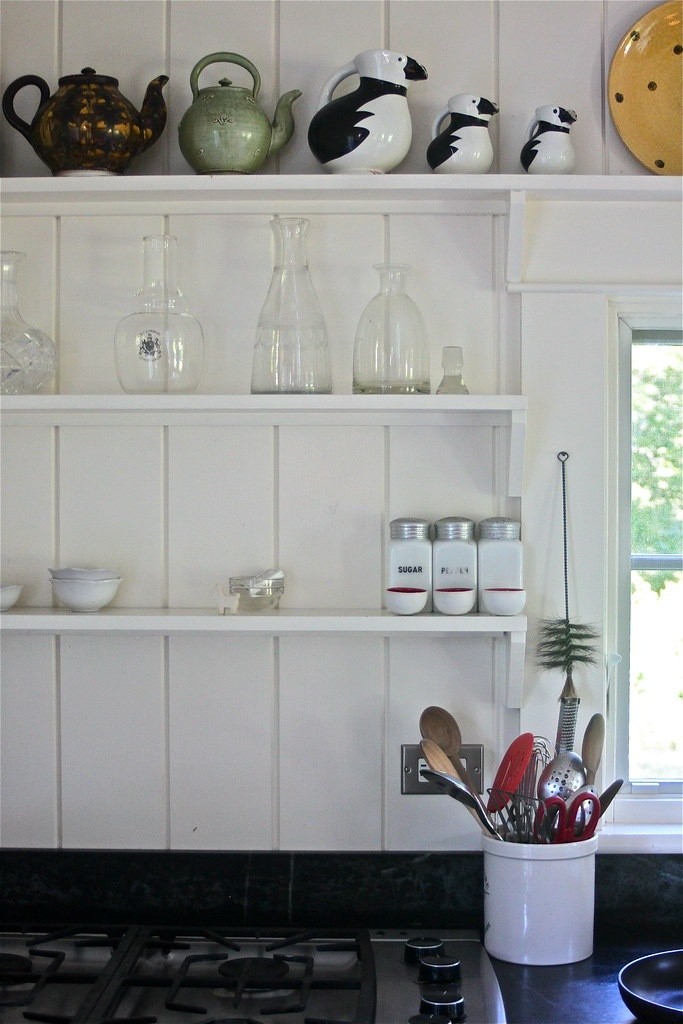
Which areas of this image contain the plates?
[606,0,683,175]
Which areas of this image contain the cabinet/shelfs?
[0,156,530,632]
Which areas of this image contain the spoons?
[419,769,505,842]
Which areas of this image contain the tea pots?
[177,51,303,175]
[1,67,170,176]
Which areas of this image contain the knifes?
[597,778,623,817]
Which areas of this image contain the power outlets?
[400,743,485,795]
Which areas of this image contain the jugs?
[425,93,499,175]
[308,50,430,178]
[519,105,577,175]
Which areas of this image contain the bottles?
[250,216,334,395]
[0,249,58,395]
[477,517,523,614]
[112,233,204,396]
[431,517,478,614]
[436,346,470,395]
[351,261,434,396]
[386,518,433,615]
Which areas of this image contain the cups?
[480,830,600,966]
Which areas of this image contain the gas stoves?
[0,922,509,1024]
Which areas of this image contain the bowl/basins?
[47,567,124,614]
[482,588,527,617]
[617,949,683,1024]
[433,588,475,615]
[229,575,284,613]
[385,587,428,616]
[0,581,23,612]
